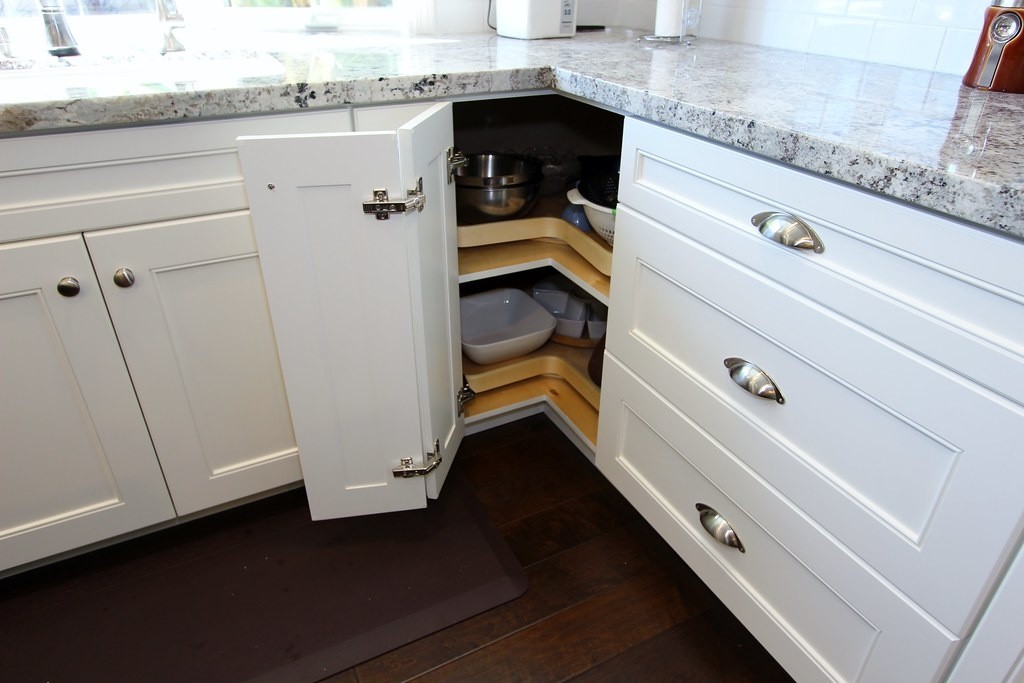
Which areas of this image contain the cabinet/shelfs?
[0,107,357,582]
[237,95,625,521]
[594,116,1024,683]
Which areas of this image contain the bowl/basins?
[455,150,545,221]
[562,203,590,233]
[459,286,559,366]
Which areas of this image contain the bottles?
[39,0,80,57]
